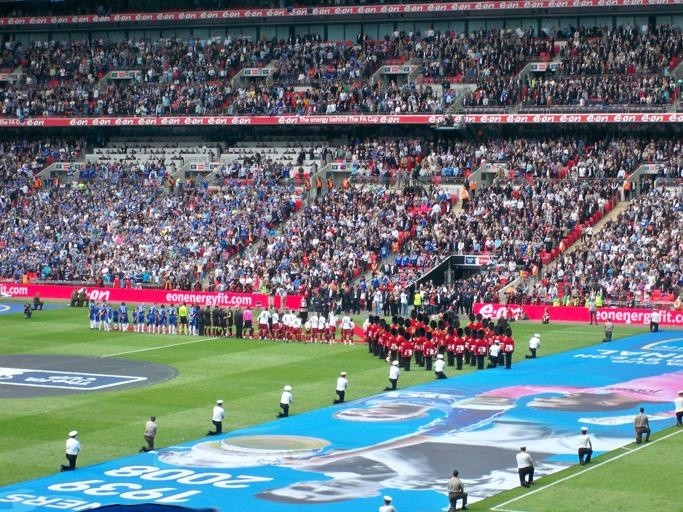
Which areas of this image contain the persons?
[156,445,282,470]
[1,0,682,113]
[138,416,157,453]
[515,446,533,488]
[634,407,650,444]
[331,371,348,404]
[206,400,225,435]
[379,495,396,512]
[478,372,682,427]
[303,386,517,461]
[0,135,683,369]
[59,430,80,471]
[276,384,292,418]
[672,391,682,427]
[577,427,592,465]
[432,354,447,379]
[446,471,467,512]
[382,360,399,391]
[247,423,629,502]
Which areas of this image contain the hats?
[384,391,683,501]
[68,371,347,438]
[369,310,541,365]
